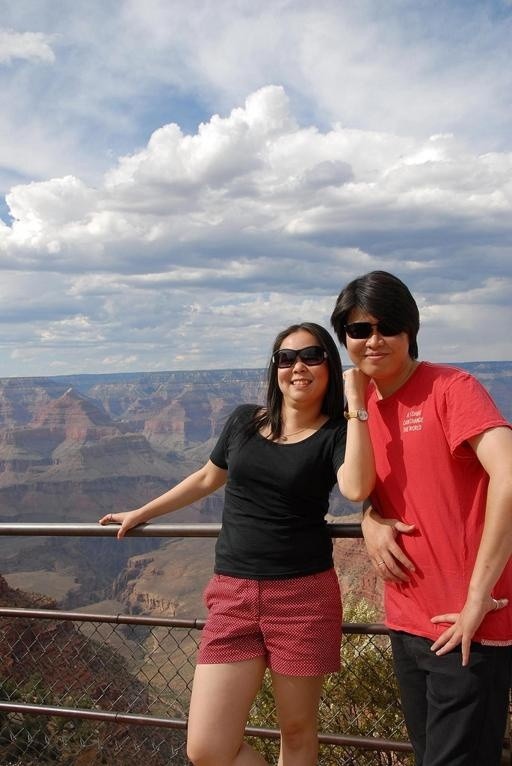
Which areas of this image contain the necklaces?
[278,416,319,441]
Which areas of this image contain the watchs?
[343,407,368,422]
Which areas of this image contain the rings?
[493,599,501,612]
[378,561,384,566]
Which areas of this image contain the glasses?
[344,320,405,339]
[273,345,330,369]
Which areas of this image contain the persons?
[331,270,511,766]
[98,321,377,765]
